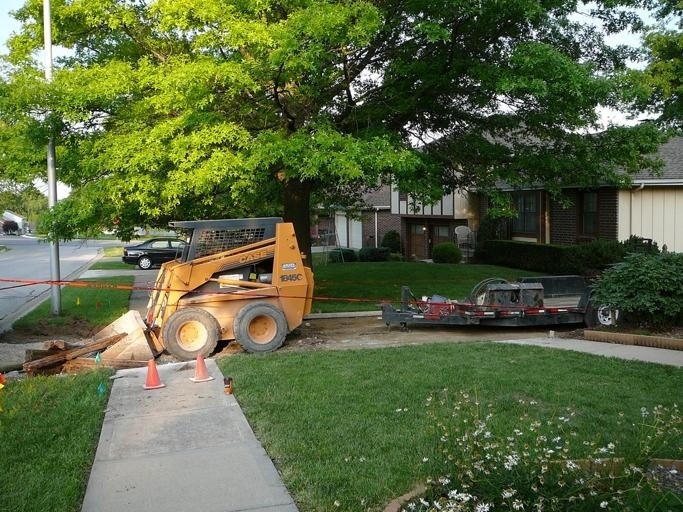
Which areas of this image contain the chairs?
[455,226,472,248]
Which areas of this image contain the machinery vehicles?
[83,215,320,369]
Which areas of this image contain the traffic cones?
[141,357,165,391]
[188,353,216,385]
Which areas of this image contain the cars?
[122,237,190,270]
[100,228,119,235]
[310,228,334,246]
[130,227,146,240]
